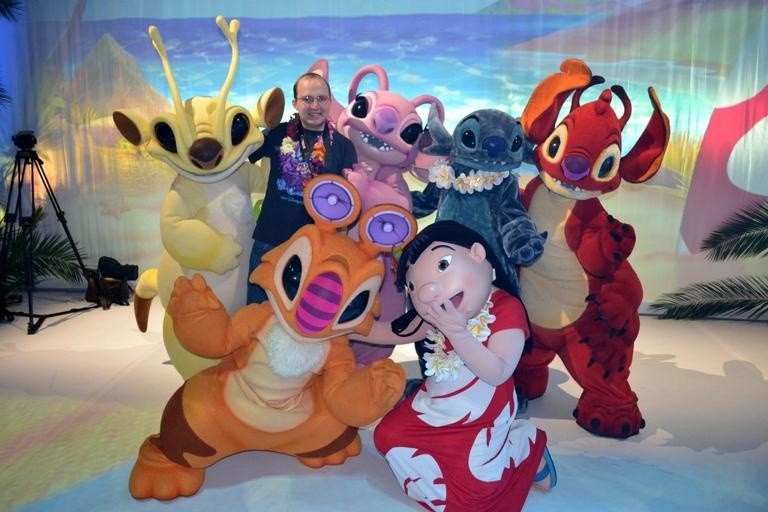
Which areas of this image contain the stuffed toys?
[126,170,422,501]
[407,102,549,294]
[311,58,454,375]
[498,57,671,438]
[110,16,287,384]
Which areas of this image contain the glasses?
[296,96,329,105]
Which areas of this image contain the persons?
[245,72,360,305]
[372,218,557,511]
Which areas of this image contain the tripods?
[0,149,105,334]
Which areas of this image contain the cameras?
[12,131,37,148]
